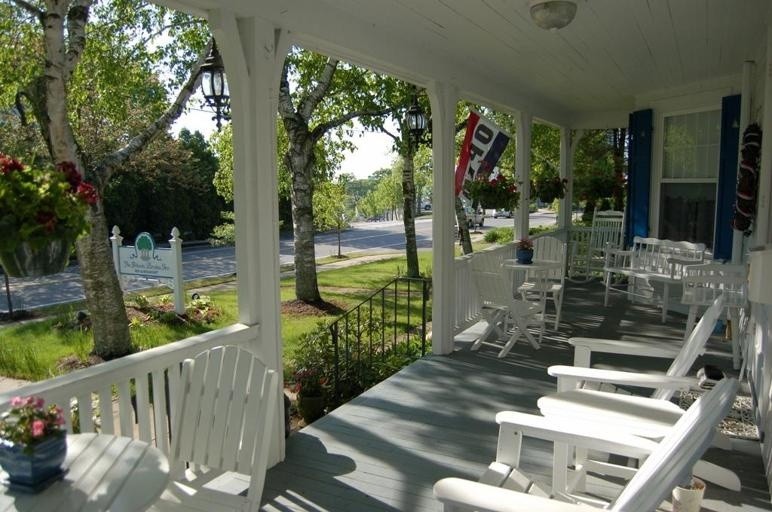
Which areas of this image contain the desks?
[0,431,171,512]
[537,386,688,498]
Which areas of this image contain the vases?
[0,233,68,278]
[0,432,71,494]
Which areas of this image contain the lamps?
[525,0,578,32]
[407,94,430,152]
[198,36,232,132]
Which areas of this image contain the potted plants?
[530,176,570,203]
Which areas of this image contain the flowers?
[0,394,67,455]
[0,151,100,259]
[462,162,521,212]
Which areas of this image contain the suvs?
[458,205,484,229]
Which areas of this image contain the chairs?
[536,286,733,467]
[430,376,743,512]
[471,205,749,371]
[140,342,281,512]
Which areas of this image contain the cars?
[416,197,433,210]
[492,209,512,219]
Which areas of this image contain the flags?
[455,106,513,208]
